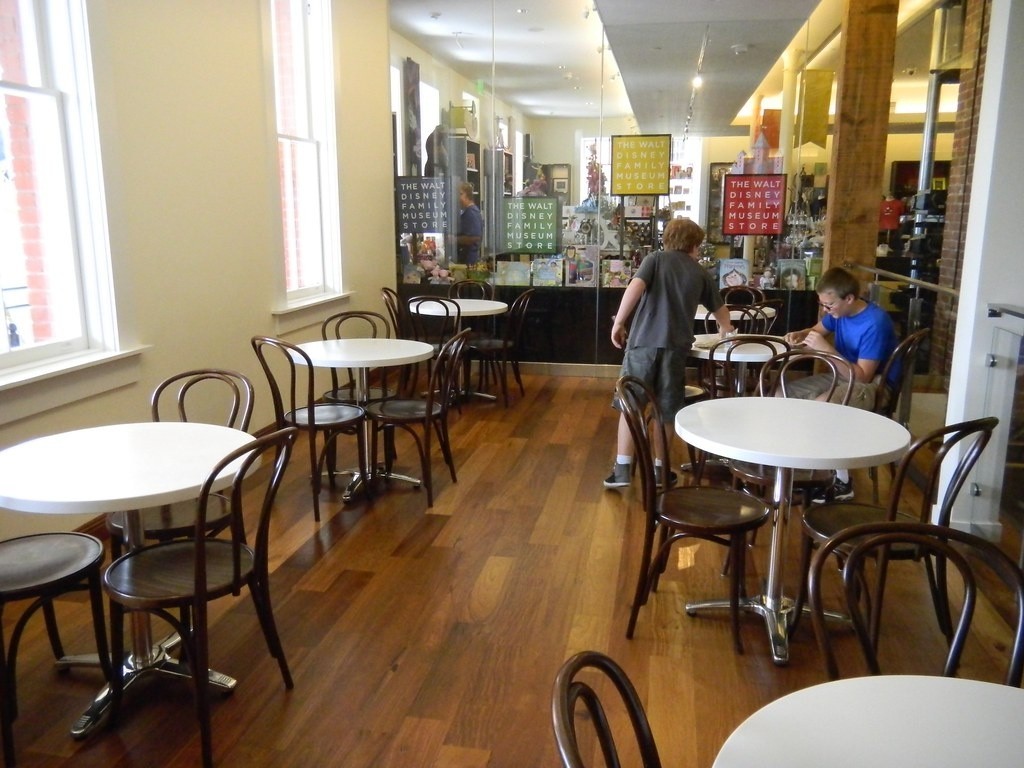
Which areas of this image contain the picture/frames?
[552,177,568,193]
[933,178,946,190]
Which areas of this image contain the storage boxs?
[804,258,822,276]
[806,275,820,291]
[778,258,806,291]
[716,258,749,290]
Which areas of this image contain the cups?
[878,245,888,257]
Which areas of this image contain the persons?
[445,182,483,262]
[783,267,800,290]
[539,261,559,272]
[809,194,827,215]
[876,194,904,229]
[772,266,899,506]
[604,217,735,488]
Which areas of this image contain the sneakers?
[811,477,855,505]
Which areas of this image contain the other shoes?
[654,465,678,488]
[603,462,632,489]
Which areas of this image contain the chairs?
[552,287,1024,768]
[0,278,536,768]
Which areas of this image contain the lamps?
[581,5,598,18]
[597,44,612,53]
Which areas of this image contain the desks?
[0,423,263,740]
[681,333,802,474]
[693,304,775,392]
[410,298,509,408]
[673,397,911,666]
[286,337,435,504]
[711,675,1024,768]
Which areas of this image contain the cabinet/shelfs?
[543,164,572,206]
[707,162,736,245]
[483,148,514,252]
[452,139,481,213]
